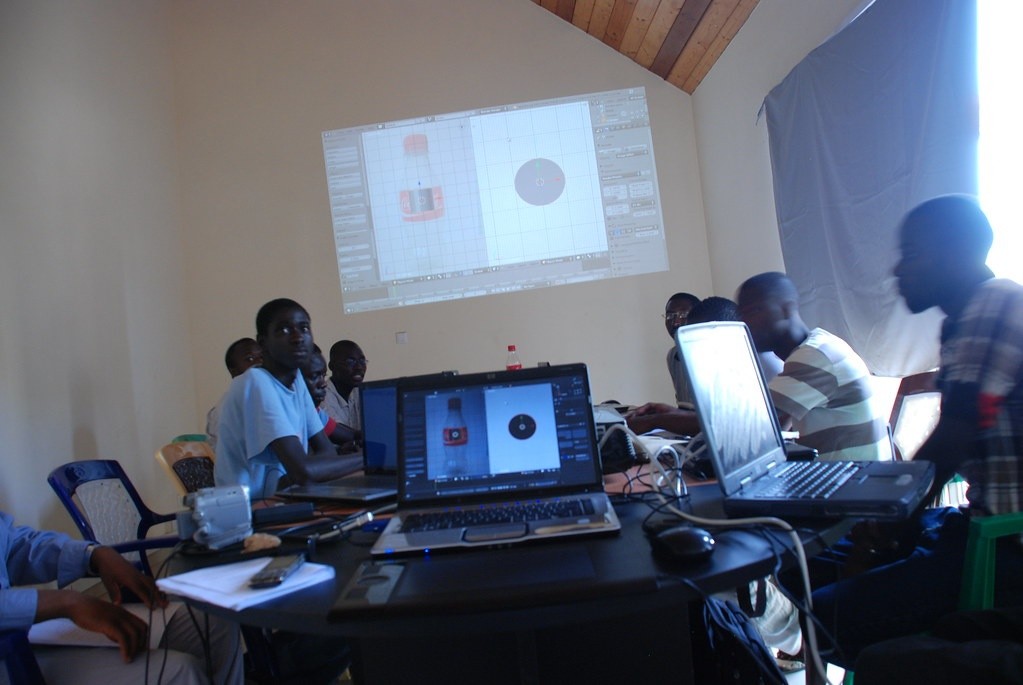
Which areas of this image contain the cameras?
[183,485,253,550]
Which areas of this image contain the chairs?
[887,372,942,461]
[46,459,185,605]
[154,441,215,497]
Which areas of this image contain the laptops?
[283,370,457,508]
[671,441,819,478]
[676,321,936,522]
[368,363,621,560]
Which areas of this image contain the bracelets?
[84,544,101,577]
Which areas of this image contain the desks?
[166,428,857,685]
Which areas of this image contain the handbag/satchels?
[735,574,807,673]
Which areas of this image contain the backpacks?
[700,592,787,685]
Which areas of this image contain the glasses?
[661,311,689,321]
[332,358,368,367]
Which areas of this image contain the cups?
[175,510,198,540]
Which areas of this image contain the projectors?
[591,406,638,473]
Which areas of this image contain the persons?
[624,271,882,673]
[772,187,1022,674]
[0,511,246,685]
[203,299,372,685]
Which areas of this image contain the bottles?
[507,344,523,370]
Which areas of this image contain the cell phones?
[248,553,306,589]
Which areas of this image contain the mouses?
[652,526,715,560]
